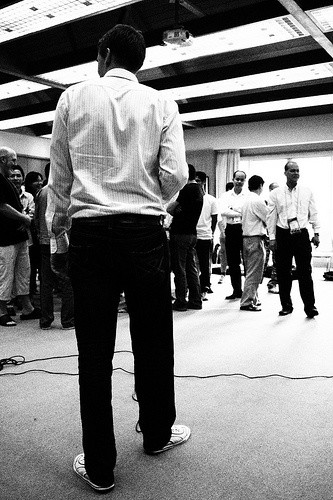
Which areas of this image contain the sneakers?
[153,425,191,453]
[73,453,115,492]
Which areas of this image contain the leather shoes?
[225,291,243,299]
[240,304,262,311]
[307,309,318,318]
[279,308,290,315]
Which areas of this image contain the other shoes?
[172,303,187,311]
[20,308,42,319]
[42,325,52,330]
[187,301,202,309]
[62,325,75,330]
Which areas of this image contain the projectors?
[162,29,194,47]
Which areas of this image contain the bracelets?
[315,233,319,236]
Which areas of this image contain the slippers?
[0,314,16,326]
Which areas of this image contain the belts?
[74,214,160,225]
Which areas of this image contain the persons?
[0,146,74,330]
[218,170,281,311]
[160,163,218,311]
[268,161,321,316]
[44,26,191,492]
